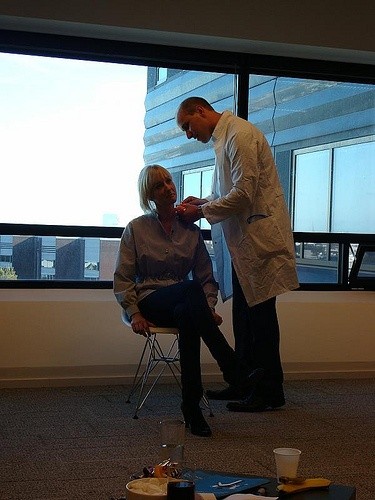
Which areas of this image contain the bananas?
[276,478,331,496]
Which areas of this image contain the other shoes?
[227,393,286,412]
[206,386,247,399]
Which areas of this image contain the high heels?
[181,402,212,437]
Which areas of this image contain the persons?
[175,96,300,412]
[114,164,261,438]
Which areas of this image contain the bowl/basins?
[125,478,176,500]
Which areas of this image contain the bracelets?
[196,206,203,218]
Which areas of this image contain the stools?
[121,304,213,420]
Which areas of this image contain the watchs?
[211,307,215,311]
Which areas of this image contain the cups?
[159,419,186,463]
[273,448,302,484]
[166,460,197,500]
